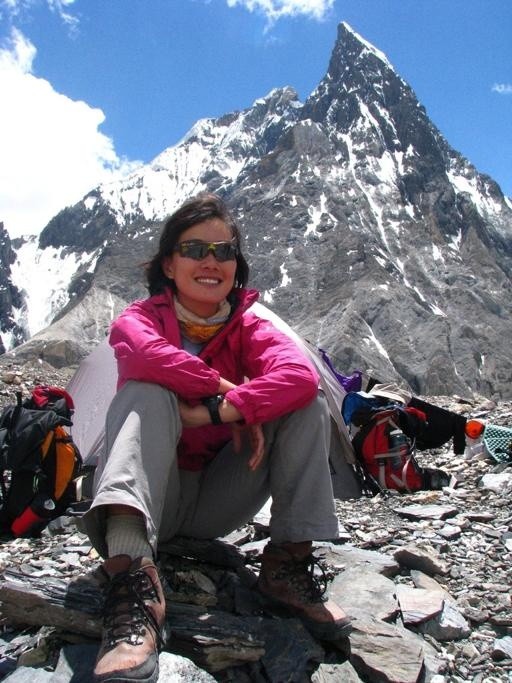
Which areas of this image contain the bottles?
[11,497,57,537]
[388,429,411,468]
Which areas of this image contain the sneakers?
[93,555,170,683]
[255,543,352,641]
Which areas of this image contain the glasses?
[170,240,237,263]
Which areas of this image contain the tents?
[63,298,361,501]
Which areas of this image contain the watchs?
[202,393,224,425]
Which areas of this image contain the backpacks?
[0,387,84,537]
[352,410,424,491]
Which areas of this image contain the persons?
[82,195,350,683]
